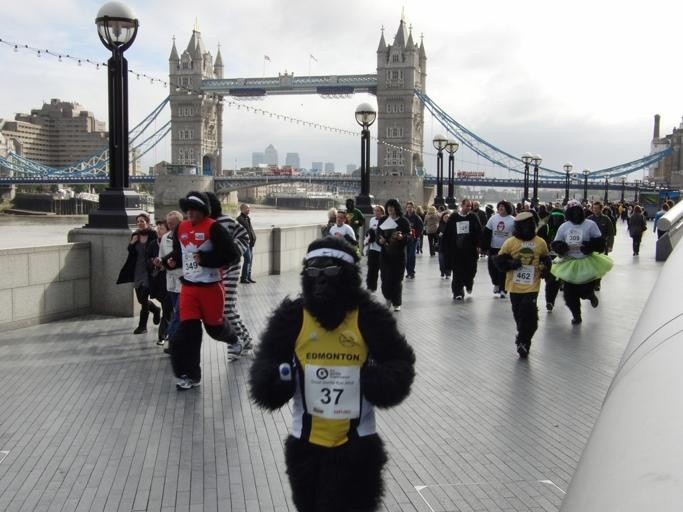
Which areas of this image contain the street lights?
[619,174,626,203]
[562,163,572,204]
[107,2,151,210]
[633,176,670,205]
[581,168,590,204]
[443,138,459,209]
[354,103,381,214]
[603,172,611,205]
[531,154,542,207]
[432,136,448,211]
[520,151,532,203]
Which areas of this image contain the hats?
[177,190,212,217]
[513,211,535,223]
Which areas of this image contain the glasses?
[303,264,344,277]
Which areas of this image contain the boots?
[147,300,161,326]
[132,310,150,336]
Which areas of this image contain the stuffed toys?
[246,237,417,512]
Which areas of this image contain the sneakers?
[441,276,450,281]
[493,289,507,299]
[455,294,462,301]
[174,376,201,391]
[383,299,399,312]
[466,288,473,293]
[239,275,255,284]
[514,339,528,354]
[545,302,553,311]
[570,314,582,325]
[229,336,245,363]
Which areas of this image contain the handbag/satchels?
[146,267,168,299]
